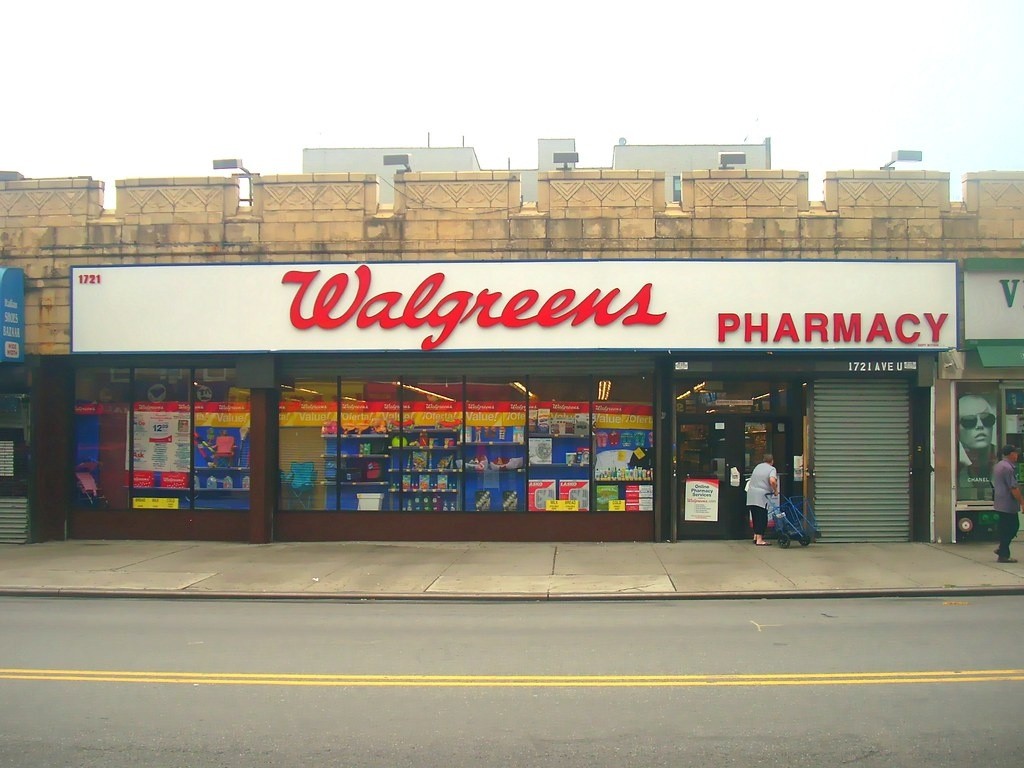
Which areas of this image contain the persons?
[958,394,999,500]
[746,453,779,545]
[993,445,1024,563]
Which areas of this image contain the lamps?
[212,150,922,178]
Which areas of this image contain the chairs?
[278,461,319,510]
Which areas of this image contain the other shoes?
[997,557,1018,563]
[994,545,1000,555]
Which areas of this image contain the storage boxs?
[529,477,652,511]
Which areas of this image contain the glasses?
[959,412,995,429]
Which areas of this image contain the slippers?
[756,541,772,546]
[754,535,766,544]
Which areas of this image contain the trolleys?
[764,490,822,547]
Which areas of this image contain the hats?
[1003,444,1021,455]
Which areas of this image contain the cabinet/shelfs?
[592,432,653,511]
[526,432,591,511]
[463,441,526,512]
[320,433,390,511]
[388,428,462,511]
[193,466,251,492]
[528,434,590,467]
